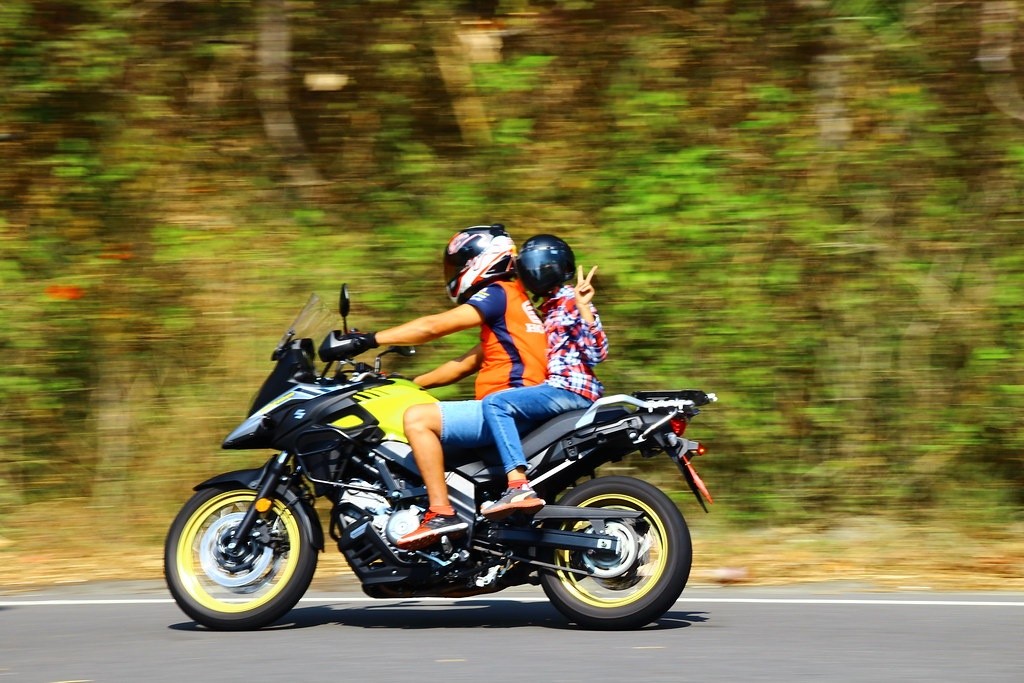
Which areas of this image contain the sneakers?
[396,509,468,551]
[482,487,546,519]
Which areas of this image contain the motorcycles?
[164,284,717,632]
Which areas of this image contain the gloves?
[339,328,379,358]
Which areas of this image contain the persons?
[480,234,610,515]
[337,224,546,548]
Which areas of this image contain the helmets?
[443,224,518,305]
[516,233,575,297]
